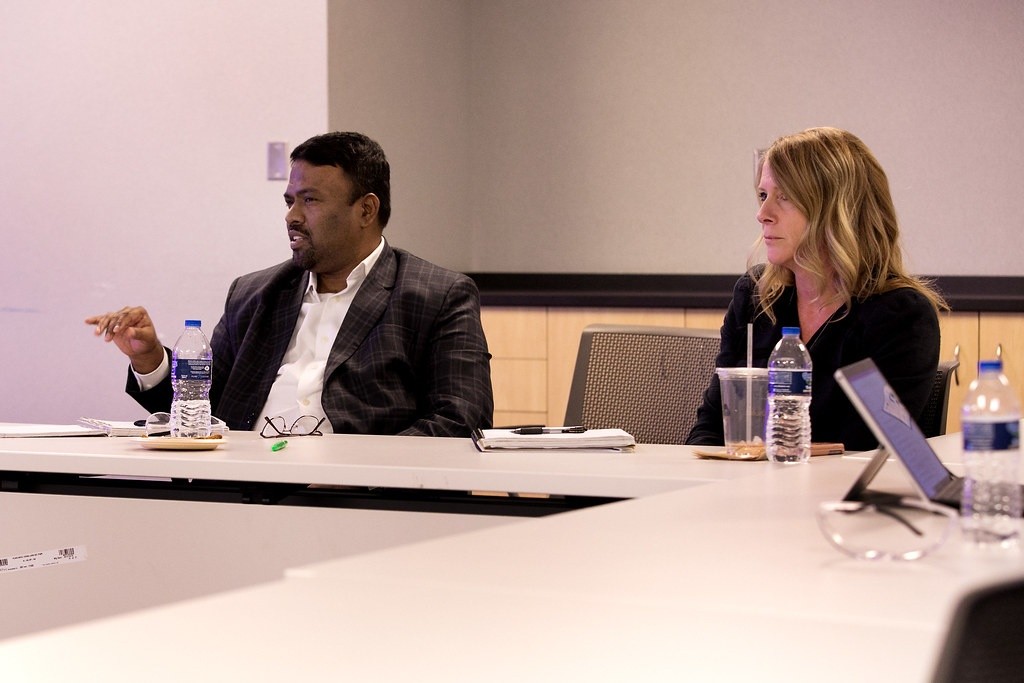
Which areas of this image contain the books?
[81,418,227,439]
[471,426,636,452]
[0,417,147,438]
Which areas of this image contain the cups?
[714,367,768,455]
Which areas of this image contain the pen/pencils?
[271,440,288,452]
[875,493,959,524]
[510,426,590,435]
[134,419,147,428]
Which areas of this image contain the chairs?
[565,322,720,445]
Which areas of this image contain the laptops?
[833,358,1024,519]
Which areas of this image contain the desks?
[0,428,1024,683]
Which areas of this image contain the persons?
[934,580,1024,683]
[85,132,493,437]
[684,127,951,445]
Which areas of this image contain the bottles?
[170,320,212,438]
[961,360,1020,548]
[765,327,813,463]
